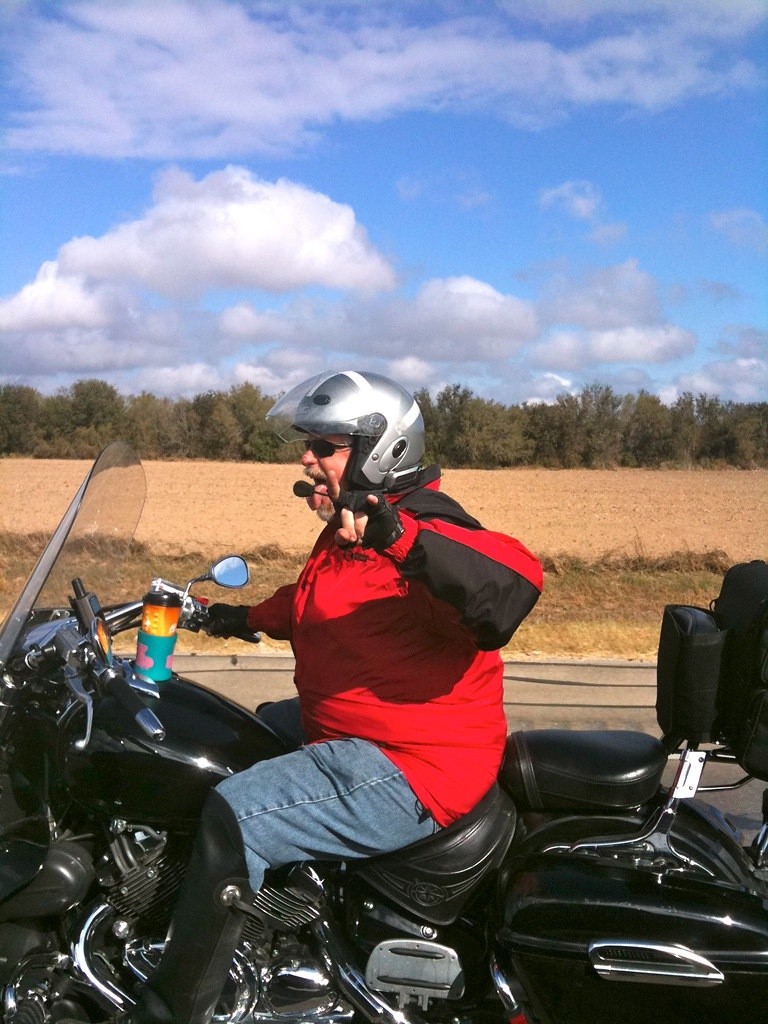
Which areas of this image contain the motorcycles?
[0,443,768,1024]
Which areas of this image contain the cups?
[135,629,178,681]
[141,591,183,636]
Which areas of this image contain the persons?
[131,370,543,1024]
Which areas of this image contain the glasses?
[302,438,355,458]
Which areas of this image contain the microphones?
[294,481,328,497]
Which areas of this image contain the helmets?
[265,370,427,494]
